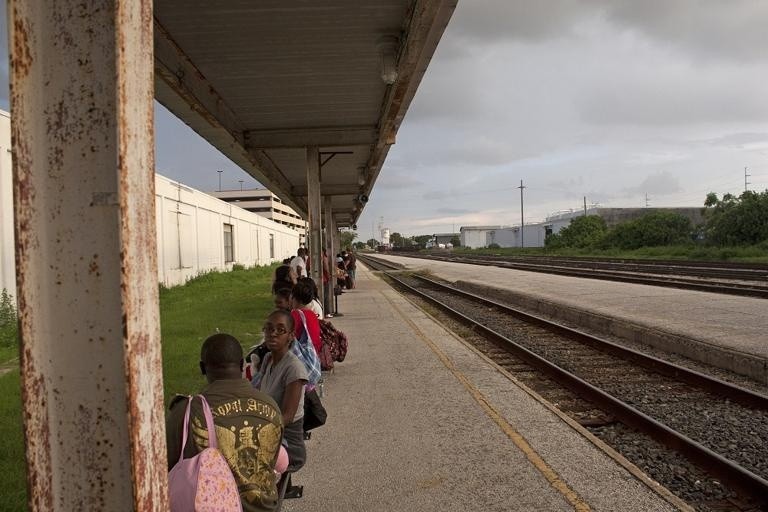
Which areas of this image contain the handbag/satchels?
[289,338,321,385]
[303,387,327,431]
[169,448,242,511]
[319,318,347,362]
[320,343,334,370]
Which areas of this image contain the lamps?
[352,199,357,212]
[357,166,366,185]
[376,36,398,86]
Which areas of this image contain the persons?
[238,244,358,391]
[252,309,309,476]
[164,331,284,511]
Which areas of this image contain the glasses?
[261,326,287,336]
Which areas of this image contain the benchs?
[277,431,311,512]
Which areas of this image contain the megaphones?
[359,193,369,203]
